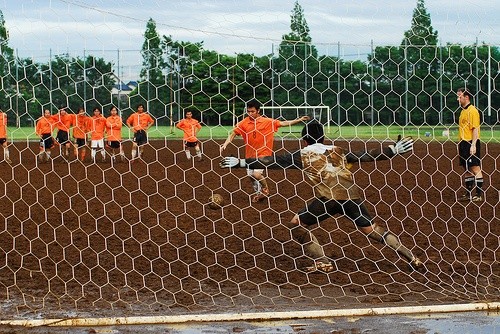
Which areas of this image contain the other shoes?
[470,195,483,202]
[259,186,269,199]
[251,195,260,203]
[408,259,424,272]
[301,261,334,273]
[458,195,473,201]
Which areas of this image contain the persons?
[220,101,310,202]
[0,112,12,164]
[456,88,484,202]
[175,110,203,161]
[219,122,421,274]
[35,104,155,164]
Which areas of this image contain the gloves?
[388,135,414,156]
[219,157,247,169]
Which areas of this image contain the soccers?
[209,194,224,210]
[443,131,448,137]
